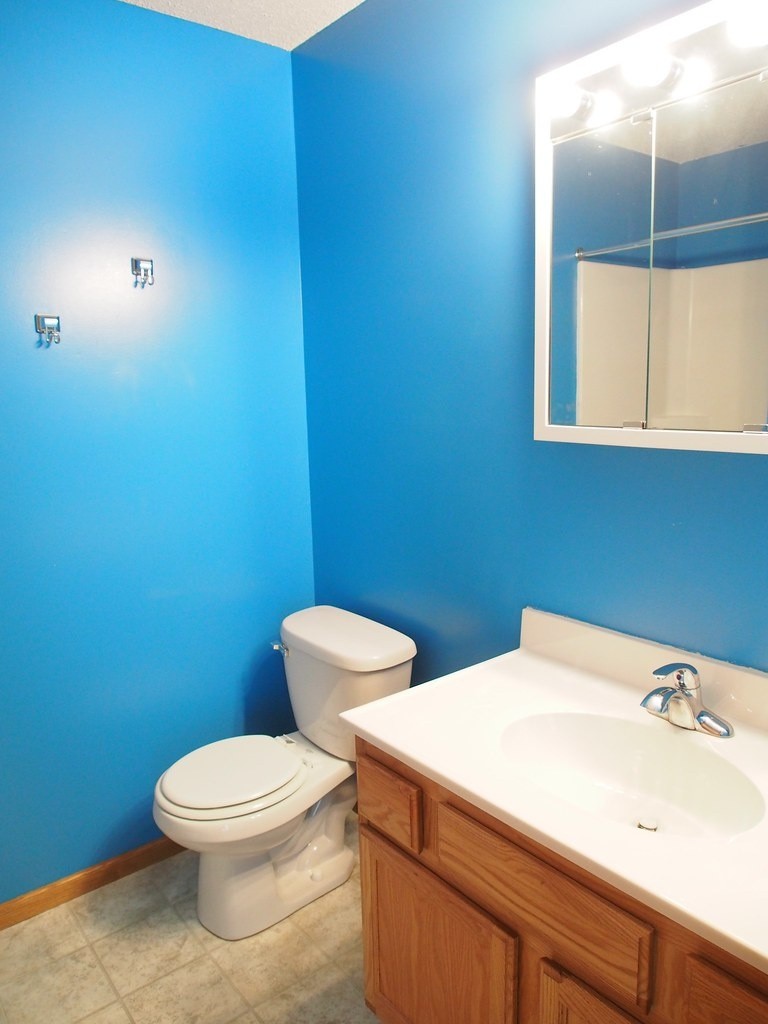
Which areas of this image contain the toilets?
[153,605,416,941]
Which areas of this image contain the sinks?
[504,709,764,842]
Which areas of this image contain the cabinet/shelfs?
[355,736,768,1024]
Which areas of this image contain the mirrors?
[534,1,768,454]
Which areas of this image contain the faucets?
[640,663,734,738]
